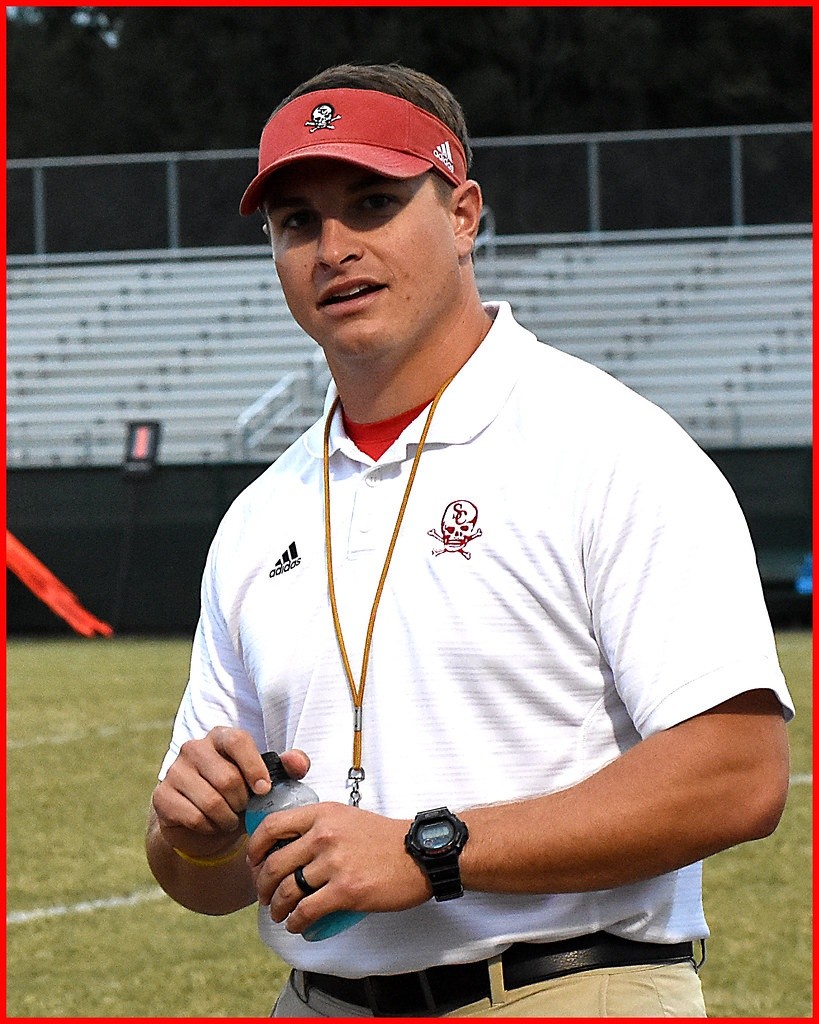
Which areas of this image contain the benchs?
[5,222,813,469]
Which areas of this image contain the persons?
[146,64,796,1021]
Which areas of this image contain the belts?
[290,940,693,1019]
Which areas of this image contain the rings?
[293,866,316,895]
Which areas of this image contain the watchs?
[402,807,470,902]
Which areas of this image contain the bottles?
[243,750,371,942]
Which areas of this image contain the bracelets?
[171,833,249,868]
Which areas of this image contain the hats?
[239,88,467,219]
